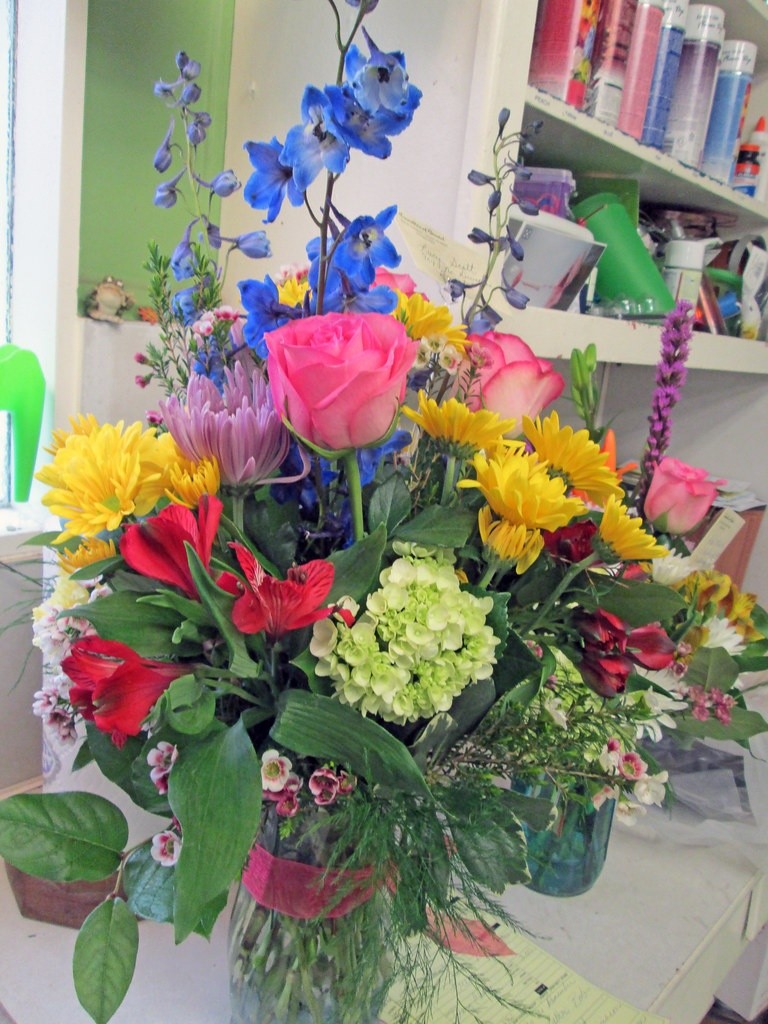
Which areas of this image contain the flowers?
[2,2,768,1023]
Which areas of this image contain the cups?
[663,240,705,328]
[517,772,616,894]
[572,192,676,313]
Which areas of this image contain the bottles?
[733,144,760,196]
[672,219,731,337]
[750,117,768,203]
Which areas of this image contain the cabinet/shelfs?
[215,0,768,1024]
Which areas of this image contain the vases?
[228,797,388,1023]
